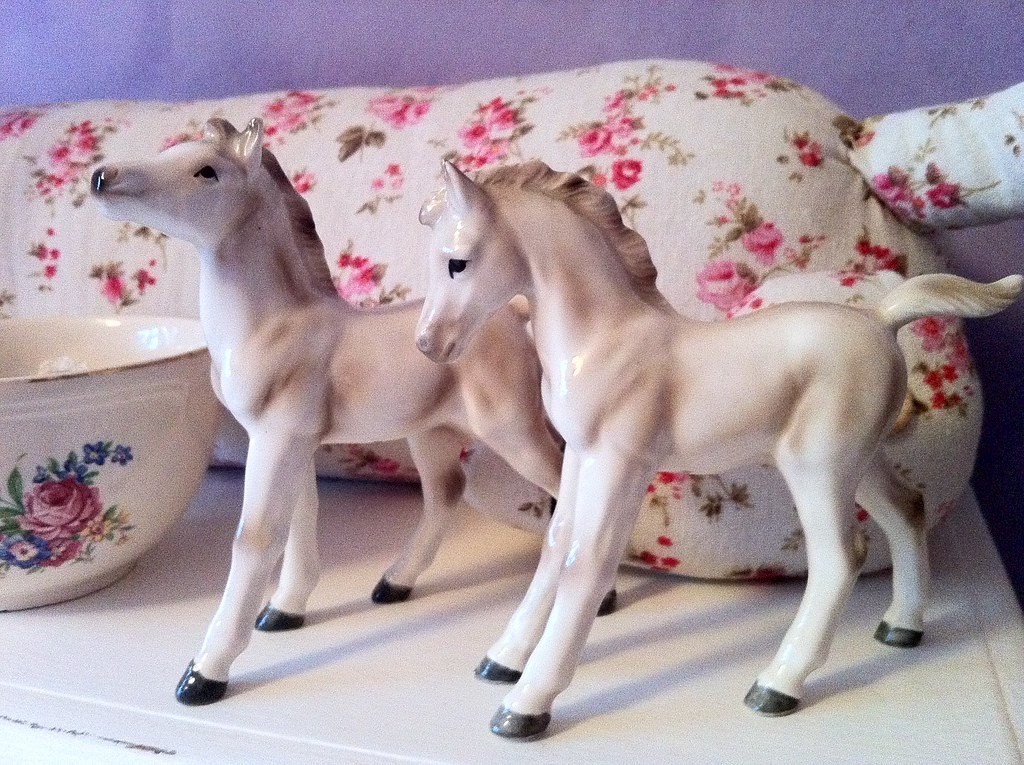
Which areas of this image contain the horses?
[90,117,1022,741]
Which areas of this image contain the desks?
[0,467,1024,765]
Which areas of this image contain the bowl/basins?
[0,315,224,613]
